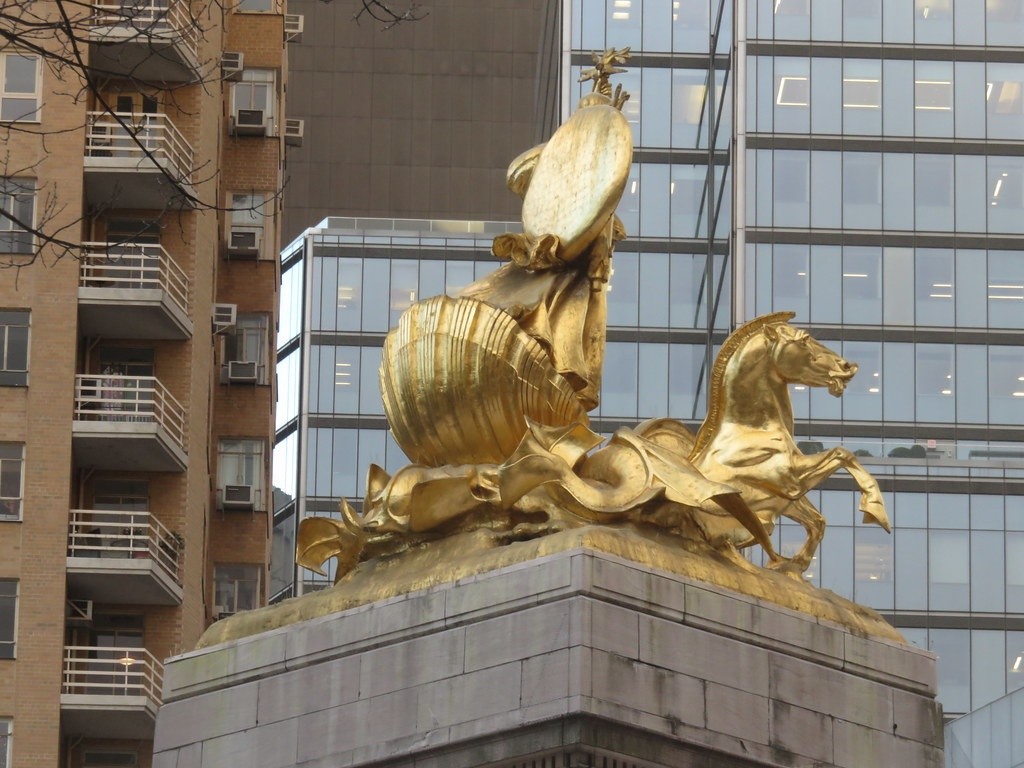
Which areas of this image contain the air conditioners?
[227,231,258,256]
[285,13,304,43]
[220,52,244,81]
[67,600,93,627]
[228,361,257,383]
[76,380,101,402]
[87,122,112,143]
[223,484,254,511]
[212,303,237,335]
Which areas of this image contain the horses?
[588,311,893,575]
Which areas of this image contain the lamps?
[237,109,266,136]
[285,119,304,147]
